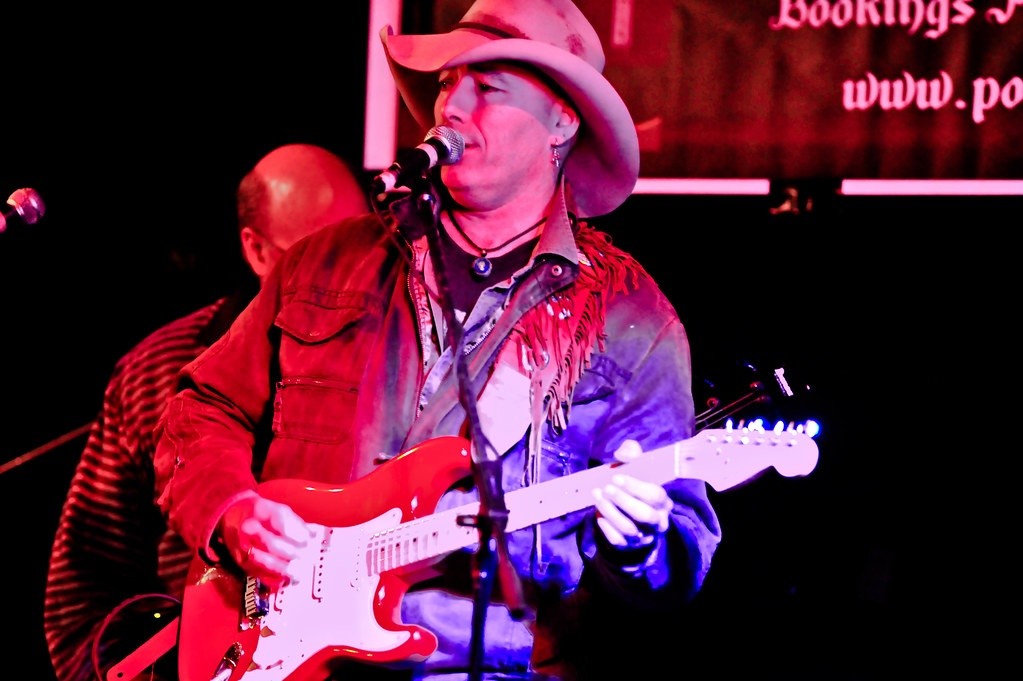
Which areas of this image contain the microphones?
[372,124,465,196]
[0,188,46,233]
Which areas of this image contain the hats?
[380,0,639,217]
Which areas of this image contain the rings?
[631,534,643,547]
[246,547,253,560]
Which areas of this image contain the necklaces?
[447,208,547,275]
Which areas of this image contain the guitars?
[175,419,822,681]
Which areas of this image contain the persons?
[151,0,721,681]
[46,144,365,681]
